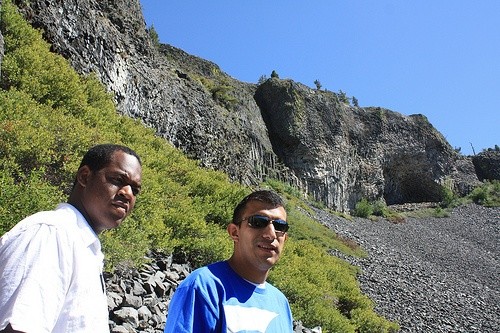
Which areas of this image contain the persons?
[0,142,144,333]
[162,190,296,333]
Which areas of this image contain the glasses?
[236,214,290,234]
